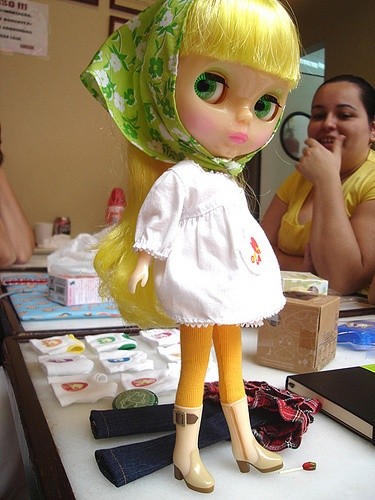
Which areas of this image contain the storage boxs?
[50,272,114,306]
[280,270,329,295]
[258,291,341,372]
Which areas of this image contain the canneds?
[52,216,70,235]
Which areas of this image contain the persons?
[0,124,35,268]
[81,0,300,493]
[257,75,375,296]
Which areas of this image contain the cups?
[34,221,54,245]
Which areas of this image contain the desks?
[0,259,375,500]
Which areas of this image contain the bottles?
[105,187,127,227]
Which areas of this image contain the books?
[286,362,375,446]
